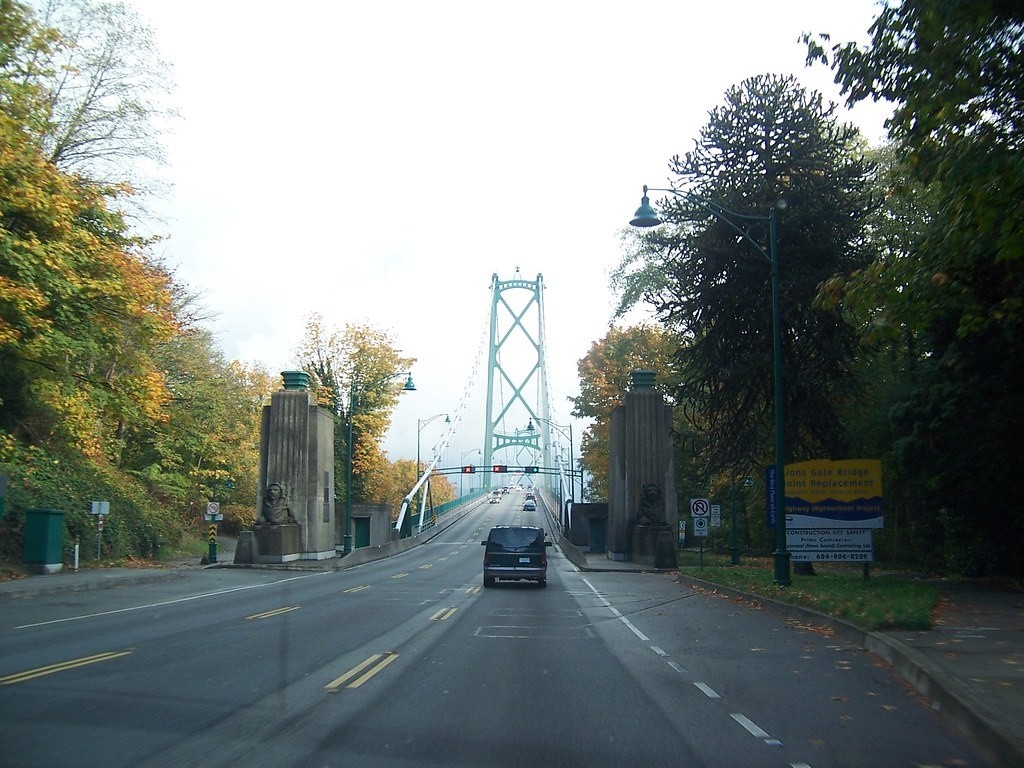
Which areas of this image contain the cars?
[487,482,538,511]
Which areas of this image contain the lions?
[254,482,296,525]
[634,483,669,527]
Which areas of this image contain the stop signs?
[98,514,104,531]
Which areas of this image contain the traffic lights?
[493,465,508,472]
[525,466,539,473]
[461,466,475,473]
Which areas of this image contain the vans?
[480,524,553,588]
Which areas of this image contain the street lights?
[459,448,483,504]
[207,463,237,563]
[627,183,793,590]
[526,417,575,530]
[545,440,571,495]
[416,413,452,529]
[479,455,496,490]
[340,372,418,557]
[729,466,756,565]
